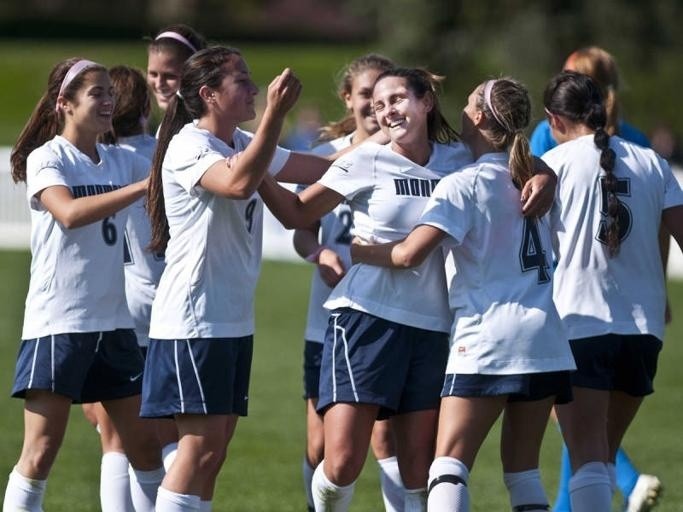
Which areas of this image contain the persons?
[3,25,681,509]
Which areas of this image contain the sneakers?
[626,470,662,512]
